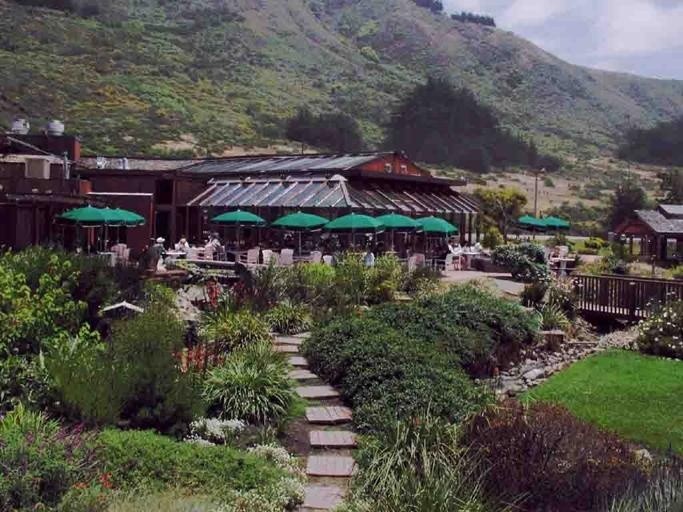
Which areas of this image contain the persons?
[178,235,189,254]
[54,231,62,243]
[323,239,333,252]
[153,237,168,254]
[271,233,293,253]
[432,239,472,270]
[205,234,221,255]
[373,243,384,256]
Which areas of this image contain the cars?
[71,234,112,255]
[258,225,452,259]
[190,227,226,245]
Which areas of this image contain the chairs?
[408,242,561,272]
[116,242,333,267]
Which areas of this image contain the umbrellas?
[322,212,378,253]
[544,216,569,231]
[115,208,144,243]
[519,215,546,228]
[52,205,109,257]
[416,216,458,234]
[211,209,266,245]
[101,207,127,251]
[377,211,421,251]
[271,210,328,257]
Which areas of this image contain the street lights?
[534,167,546,216]
[619,233,627,262]
